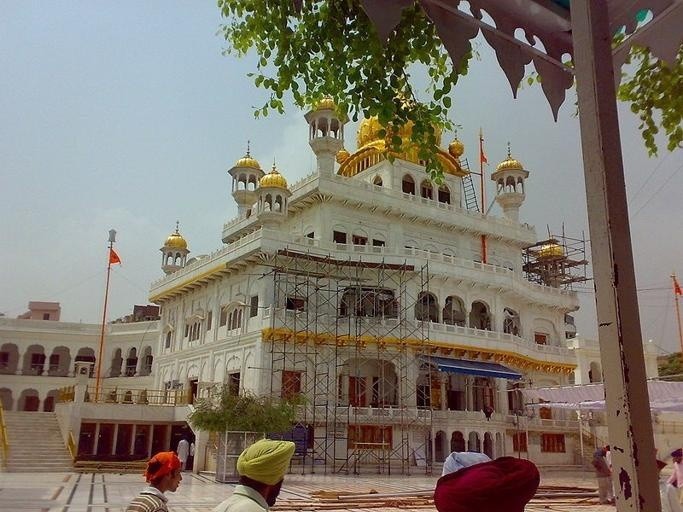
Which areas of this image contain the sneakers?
[601,497,616,506]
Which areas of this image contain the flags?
[480,141,488,166]
[673,279,682,295]
[110,248,121,265]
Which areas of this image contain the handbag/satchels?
[590,456,605,472]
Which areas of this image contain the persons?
[604,445,615,502]
[592,448,610,504]
[208,437,295,512]
[174,434,189,472]
[125,451,183,512]
[665,447,683,489]
